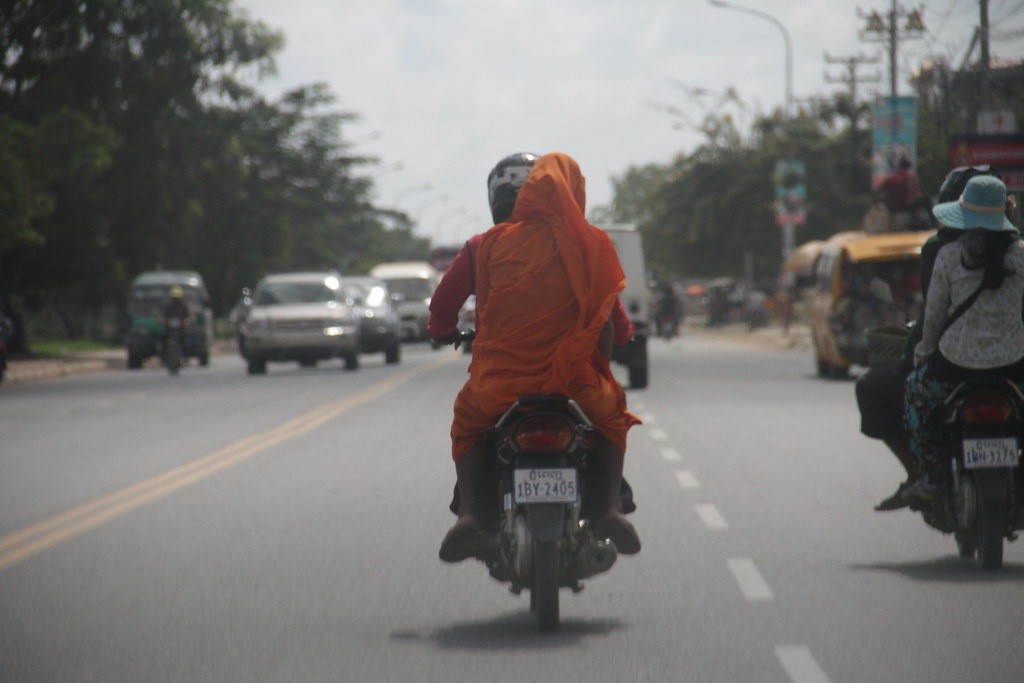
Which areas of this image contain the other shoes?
[901,478,950,501]
[874,482,910,510]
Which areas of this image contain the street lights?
[707,0,792,124]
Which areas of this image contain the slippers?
[439,523,480,561]
[594,516,640,554]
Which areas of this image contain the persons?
[857,165,1023,509]
[426,153,639,562]
[872,158,920,209]
[160,286,189,329]
[655,287,679,336]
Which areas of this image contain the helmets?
[170,286,182,298]
[487,153,540,225]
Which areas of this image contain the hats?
[932,176,1021,233]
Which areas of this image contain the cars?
[338,276,404,365]
[242,272,360,375]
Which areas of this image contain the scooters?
[161,318,185,375]
[654,308,681,340]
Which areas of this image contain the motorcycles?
[902,367,1023,572]
[431,326,629,634]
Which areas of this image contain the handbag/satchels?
[903,363,948,444]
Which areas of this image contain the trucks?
[590,224,649,388]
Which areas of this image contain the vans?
[782,227,935,383]
[123,271,213,370]
[367,260,435,343]
